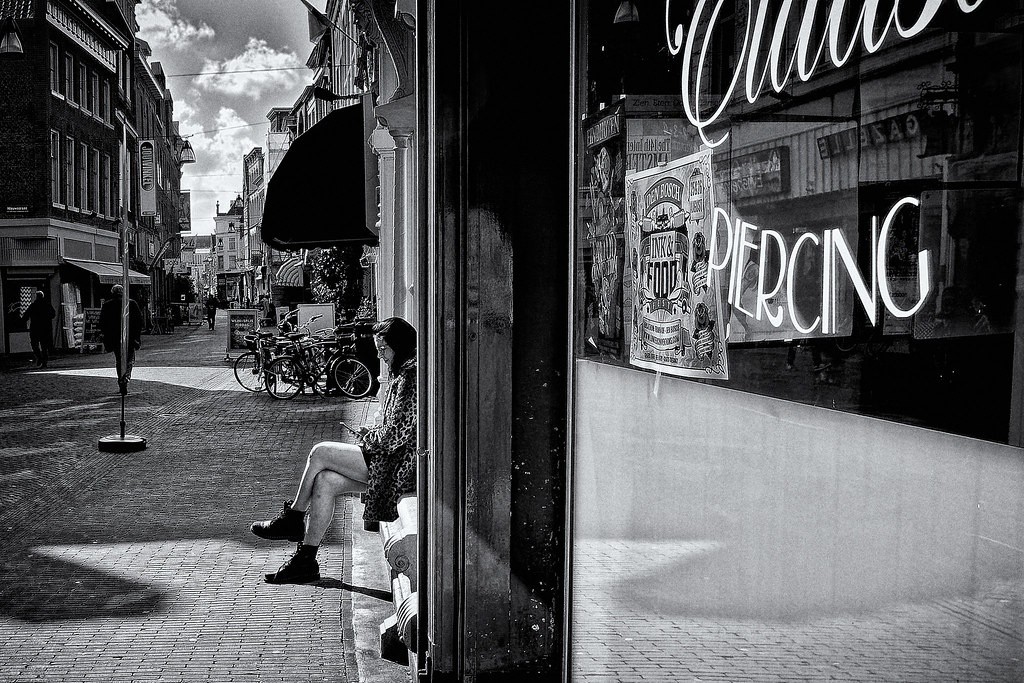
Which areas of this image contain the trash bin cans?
[354,320,380,398]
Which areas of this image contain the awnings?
[68,259,151,286]
[262,103,379,250]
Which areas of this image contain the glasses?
[376,344,388,352]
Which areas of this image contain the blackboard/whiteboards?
[80,307,106,346]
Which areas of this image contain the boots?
[264,542,320,584]
[249,499,306,542]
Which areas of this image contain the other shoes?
[37,355,43,366]
[212,323,214,330]
[209,323,212,329]
[38,362,47,370]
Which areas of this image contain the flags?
[308,10,328,45]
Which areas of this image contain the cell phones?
[339,422,364,439]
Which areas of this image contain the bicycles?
[233,308,372,400]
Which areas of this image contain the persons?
[22,290,57,369]
[98,283,144,393]
[250,318,417,585]
[205,294,218,330]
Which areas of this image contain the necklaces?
[245,297,250,308]
